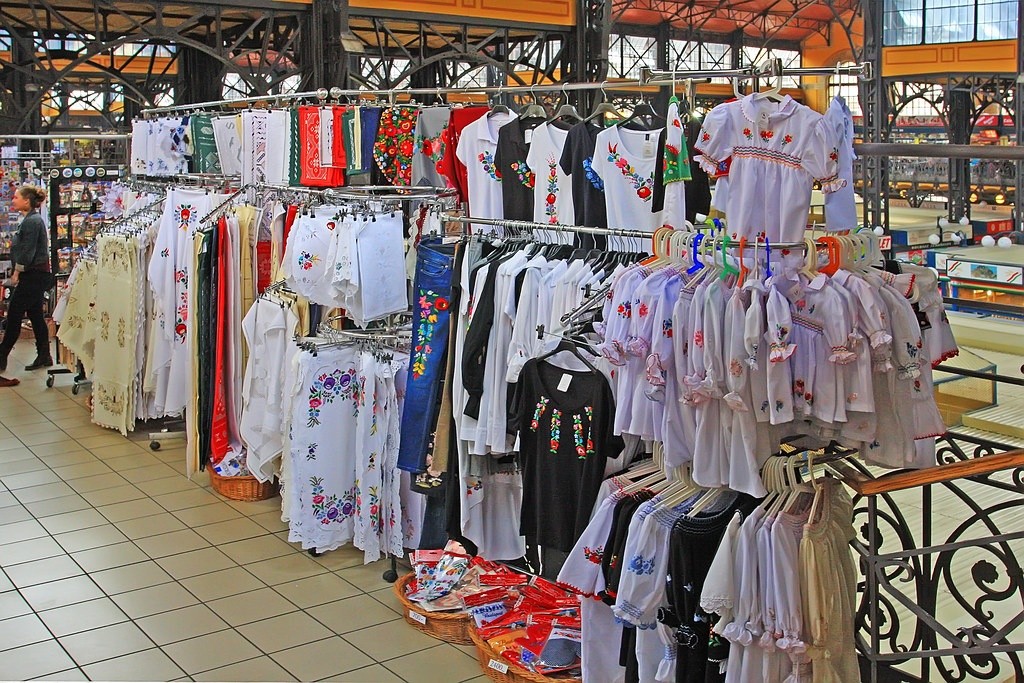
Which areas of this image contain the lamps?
[928,209,1024,249]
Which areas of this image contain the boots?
[25,341,53,370]
[0,341,15,370]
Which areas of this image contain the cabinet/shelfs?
[51,165,120,309]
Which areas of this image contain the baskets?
[393,573,477,646]
[468,620,582,683]
[206,463,279,501]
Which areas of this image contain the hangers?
[78,58,881,525]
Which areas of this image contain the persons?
[0,185,53,370]
[0,375,20,387]
